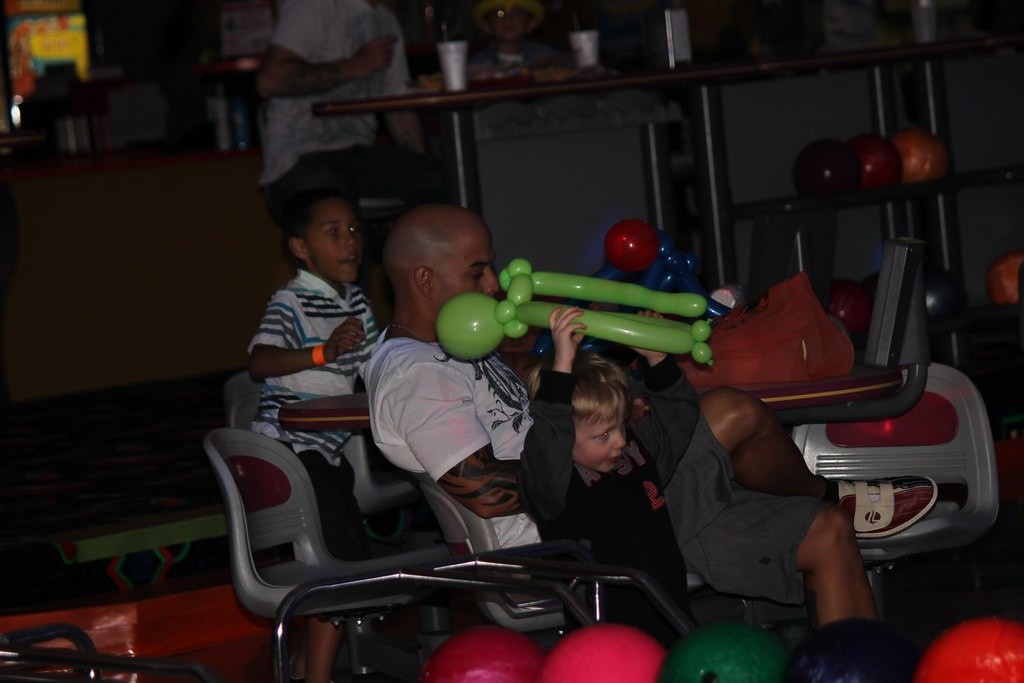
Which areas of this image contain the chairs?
[412,467,572,629]
[786,359,999,613]
[204,427,452,682]
[223,369,269,428]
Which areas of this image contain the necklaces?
[391,324,428,342]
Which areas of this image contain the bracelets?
[312,345,326,366]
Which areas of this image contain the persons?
[248,0,878,683]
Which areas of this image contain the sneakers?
[838,474,940,538]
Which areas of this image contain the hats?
[471,0,543,34]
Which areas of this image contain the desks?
[310,25,1023,373]
[279,393,418,516]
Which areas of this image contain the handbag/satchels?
[674,271,854,387]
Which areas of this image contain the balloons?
[436,259,715,368]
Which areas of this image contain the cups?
[910,0,938,43]
[436,41,469,92]
[569,30,600,67]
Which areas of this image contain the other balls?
[987,248,1024,305]
[417,627,542,683]
[912,616,1024,683]
[889,130,947,184]
[792,137,862,197]
[782,617,922,683]
[657,618,790,683]
[922,262,964,321]
[541,623,666,683]
[846,133,902,188]
[828,279,874,332]
[604,218,658,273]
